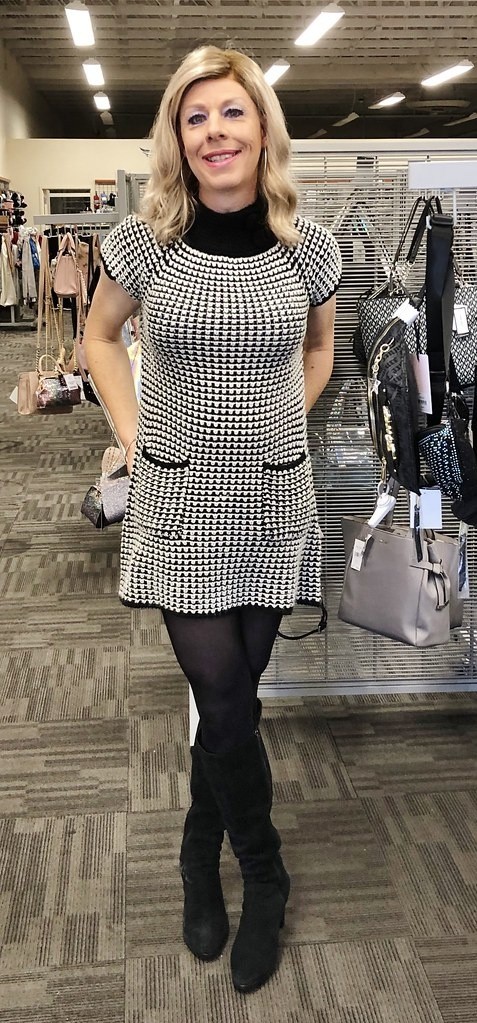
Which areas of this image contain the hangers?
[40,223,103,237]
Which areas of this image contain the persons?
[84,46,341,993]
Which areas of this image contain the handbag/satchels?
[336,516,464,647]
[349,325,368,364]
[417,424,477,504]
[358,195,477,387]
[368,309,422,496]
[100,445,130,523]
[81,483,112,528]
[18,225,140,417]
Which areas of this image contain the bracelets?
[124,439,136,463]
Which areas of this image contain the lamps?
[332,112,360,127]
[368,91,406,109]
[81,58,106,88]
[65,1,95,52]
[406,128,429,137]
[100,111,114,125]
[263,58,291,86]
[93,91,111,111]
[445,112,477,127]
[294,4,346,47]
[421,58,475,86]
[310,128,327,138]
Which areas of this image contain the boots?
[180,698,262,962]
[196,716,290,994]
